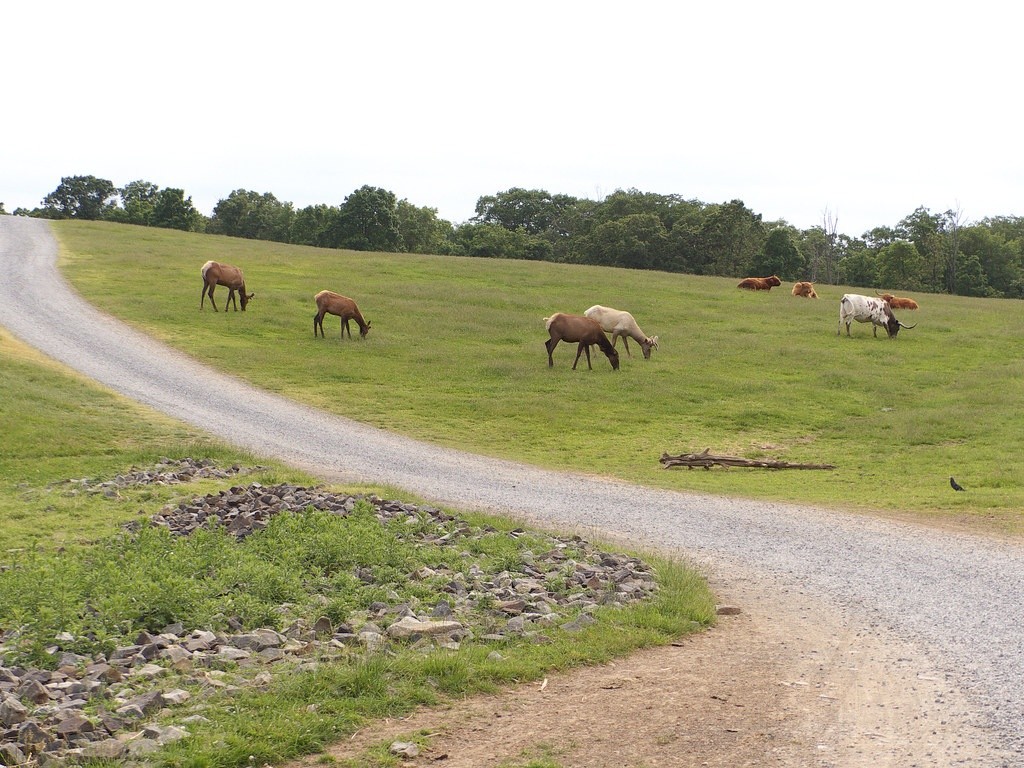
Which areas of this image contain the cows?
[838,295,917,339]
[875,289,919,310]
[792,281,817,298]
[738,274,781,291]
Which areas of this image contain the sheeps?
[584,304,660,360]
[200,260,255,312]
[544,313,620,373]
[313,290,372,341]
